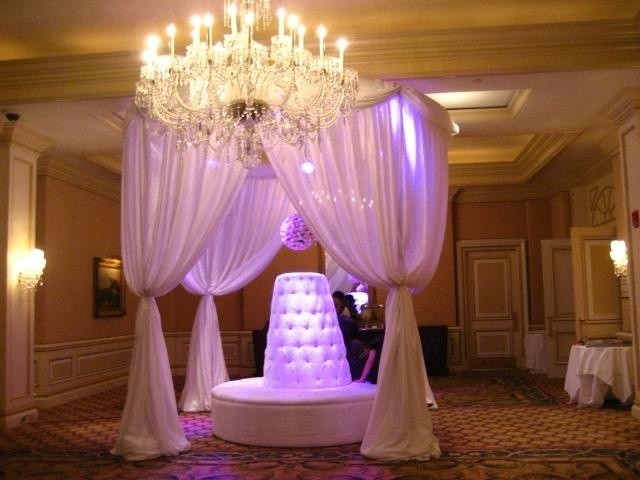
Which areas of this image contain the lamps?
[17,248,47,293]
[134,0,359,170]
[359,302,385,328]
[609,240,628,278]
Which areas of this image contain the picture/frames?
[93,255,127,318]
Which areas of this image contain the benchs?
[347,292,368,314]
[210,376,377,448]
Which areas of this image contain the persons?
[332,291,383,384]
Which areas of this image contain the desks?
[524,334,549,375]
[563,343,634,409]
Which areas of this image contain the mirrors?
[317,238,376,320]
